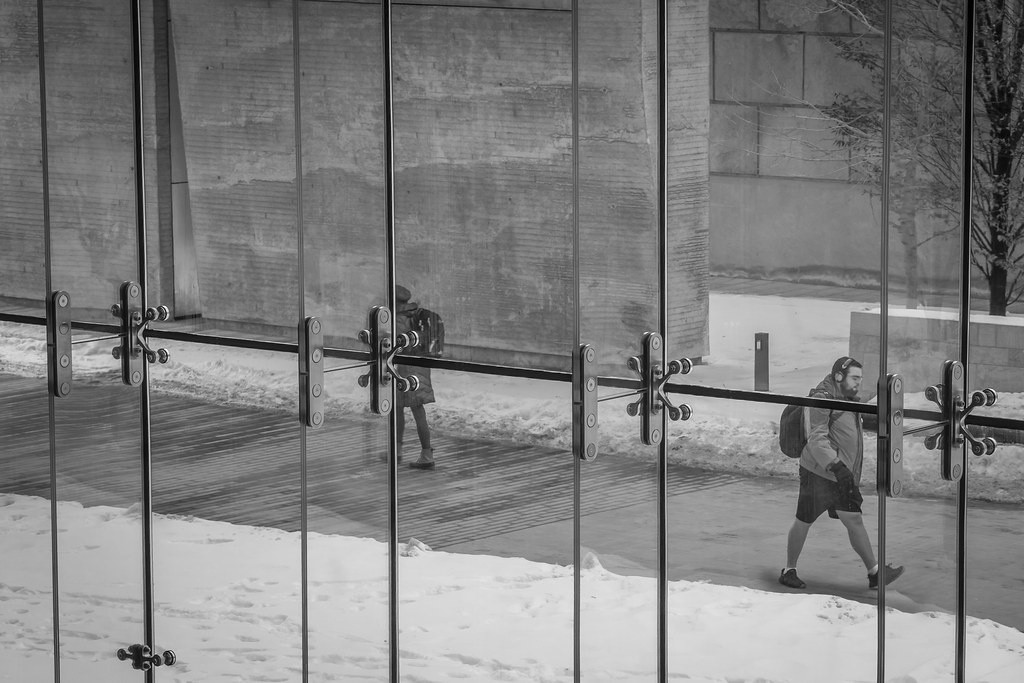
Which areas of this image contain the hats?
[396,284,412,302]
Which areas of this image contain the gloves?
[829,460,854,495]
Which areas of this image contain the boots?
[410,448,437,469]
[380,442,404,464]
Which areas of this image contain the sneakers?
[868,563,904,589]
[778,568,806,589]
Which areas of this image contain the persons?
[378,282,435,468]
[780,356,906,588]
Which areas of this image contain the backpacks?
[397,308,445,358]
[780,388,834,458]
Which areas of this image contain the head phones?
[835,360,854,383]
